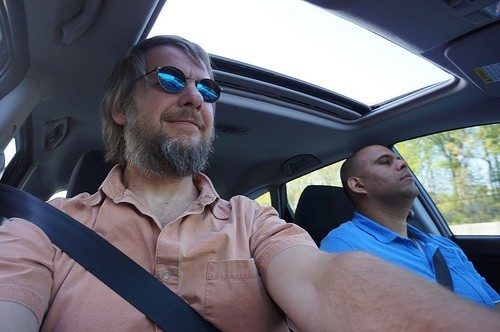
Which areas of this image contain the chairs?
[295,183,355,255]
[66,149,111,198]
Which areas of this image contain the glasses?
[134,65,224,103]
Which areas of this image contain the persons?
[318,144,500,314]
[1,35,500,332]
